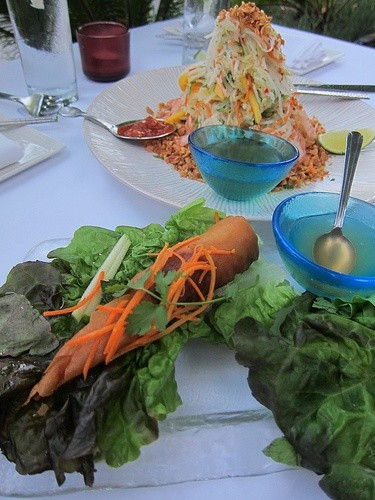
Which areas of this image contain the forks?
[0,89,73,117]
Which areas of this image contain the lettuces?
[0,201,375,499]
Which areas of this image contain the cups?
[181,0,233,66]
[76,21,131,82]
[5,0,78,107]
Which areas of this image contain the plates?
[0,120,67,185]
[284,46,344,76]
[84,65,375,221]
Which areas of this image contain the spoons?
[313,130,365,275]
[59,104,177,141]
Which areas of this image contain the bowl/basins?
[275,192,375,306]
[188,123,299,201]
